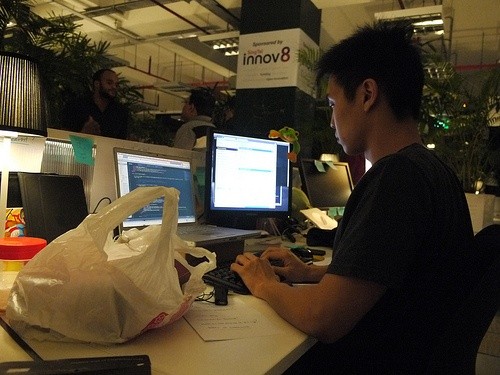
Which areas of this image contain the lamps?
[375,5,445,38]
[197,31,239,56]
[0,52,48,238]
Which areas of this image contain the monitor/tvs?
[207,130,293,239]
[299,158,355,221]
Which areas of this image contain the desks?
[0,225,351,375]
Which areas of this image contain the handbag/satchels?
[5,186,218,344]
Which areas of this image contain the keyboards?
[203,254,313,296]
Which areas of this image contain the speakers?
[16,173,88,245]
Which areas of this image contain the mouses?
[291,248,313,257]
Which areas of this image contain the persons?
[230,21,475,375]
[174,90,241,150]
[61,69,129,139]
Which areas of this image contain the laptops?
[112,148,263,246]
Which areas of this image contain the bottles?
[0,237,47,314]
